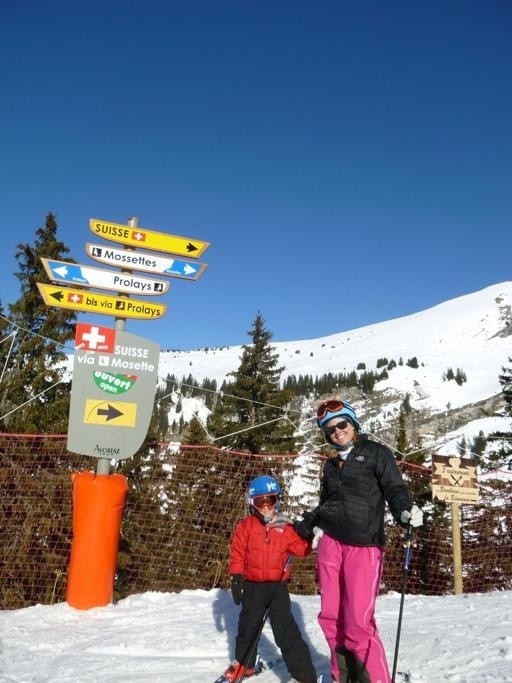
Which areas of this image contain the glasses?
[324,420,348,434]
[248,493,281,510]
[317,400,343,419]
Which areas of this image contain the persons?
[289,399,425,683]
[224,474,325,682]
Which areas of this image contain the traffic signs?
[36,280,167,326]
[90,217,210,257]
[66,319,161,459]
[40,257,169,295]
[85,241,208,280]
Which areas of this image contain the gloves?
[401,505,424,528]
[296,510,324,550]
[231,574,242,605]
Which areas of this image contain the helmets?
[247,476,281,513]
[317,400,360,428]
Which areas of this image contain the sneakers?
[224,661,256,682]
